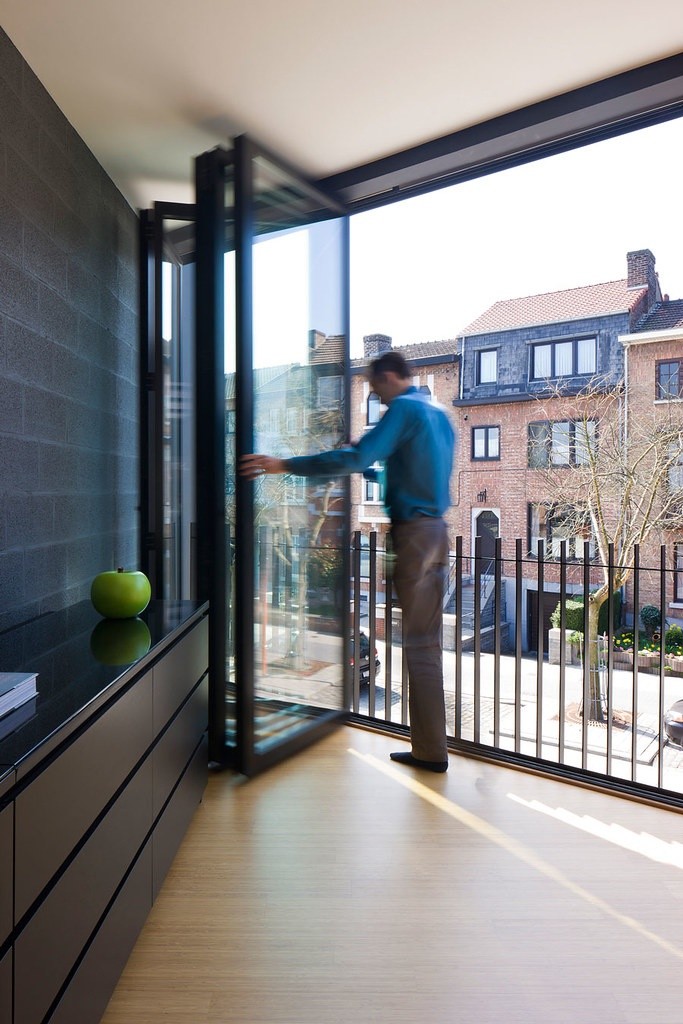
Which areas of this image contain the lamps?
[476,489,487,503]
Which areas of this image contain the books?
[0,695,40,744]
[0,672,38,719]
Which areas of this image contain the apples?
[89,616,150,664]
[91,566,151,620]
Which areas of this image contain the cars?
[258,623,383,691]
[664,696,683,750]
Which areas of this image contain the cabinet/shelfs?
[0,599,210,1024]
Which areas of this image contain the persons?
[240,353,456,772]
